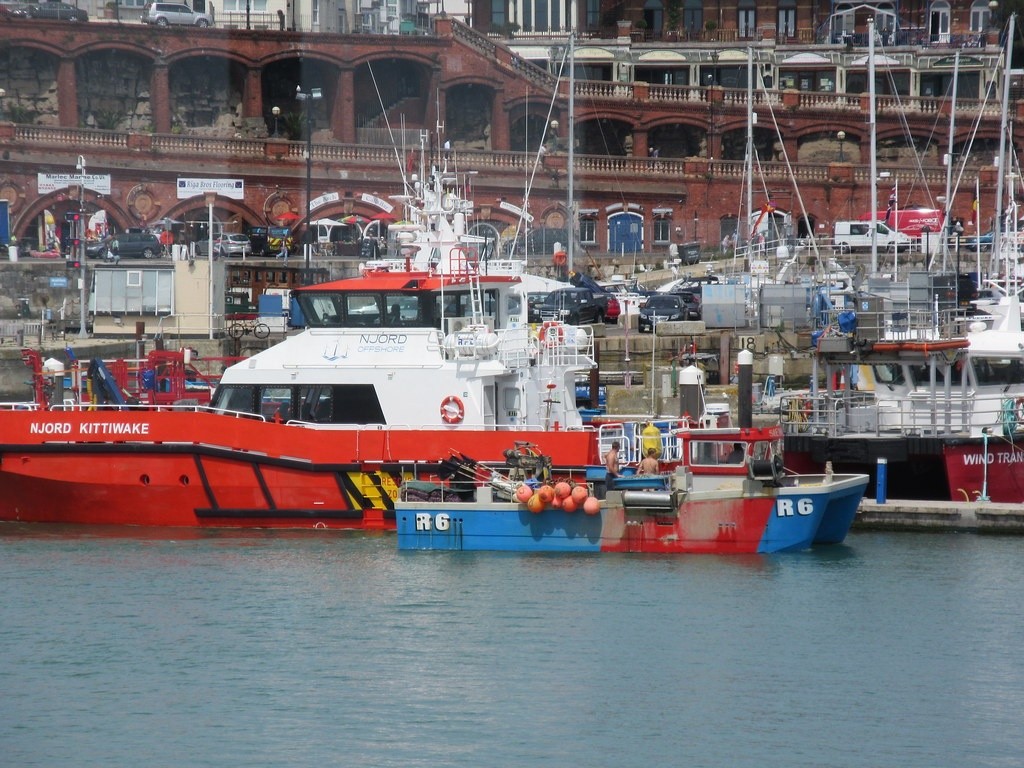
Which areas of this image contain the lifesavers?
[1015,397,1024,425]
[539,322,565,350]
[439,396,465,425]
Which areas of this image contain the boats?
[393,422,871,551]
[0,18,1024,530]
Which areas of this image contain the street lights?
[836,130,846,164]
[0,86,5,119]
[550,42,560,76]
[550,120,561,156]
[989,0,1000,30]
[270,104,282,137]
[710,49,719,84]
[292,85,324,275]
[707,73,716,158]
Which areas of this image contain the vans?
[244,225,293,256]
[833,221,913,255]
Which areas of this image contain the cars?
[0,0,89,22]
[194,231,252,256]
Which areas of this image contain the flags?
[466,178,470,192]
[407,151,414,169]
[972,184,976,222]
[212,236,221,253]
[885,186,896,221]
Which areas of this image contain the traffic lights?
[66,260,82,270]
[65,212,84,222]
[65,238,81,249]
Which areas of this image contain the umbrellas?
[372,212,399,218]
[304,218,348,228]
[273,211,302,221]
[337,215,370,223]
[395,221,412,225]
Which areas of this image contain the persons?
[15,300,31,319]
[721,231,766,257]
[951,221,965,236]
[104,232,121,264]
[60,236,68,256]
[276,237,286,259]
[605,442,744,492]
[274,399,329,425]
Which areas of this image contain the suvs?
[964,226,1024,252]
[502,226,576,256]
[86,229,163,260]
[140,2,215,28]
